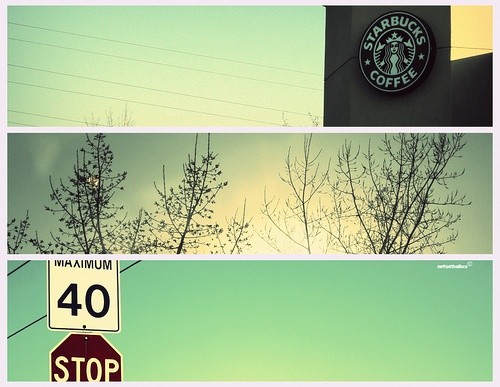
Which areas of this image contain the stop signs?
[50,334,121,382]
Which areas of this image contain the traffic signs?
[48,259,119,332]
